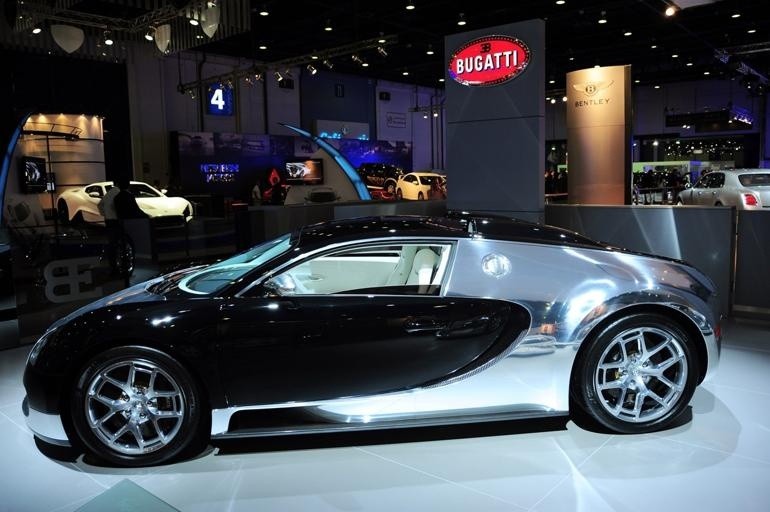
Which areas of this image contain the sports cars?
[54,179,195,233]
[20,210,729,472]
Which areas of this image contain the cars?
[395,170,450,202]
[301,186,341,205]
[351,160,405,194]
[670,164,770,211]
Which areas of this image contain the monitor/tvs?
[199,162,240,182]
[281,157,323,185]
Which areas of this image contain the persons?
[96,171,125,228]
[545,167,567,200]
[634,169,707,204]
[426,176,448,200]
[251,178,263,203]
[114,176,139,224]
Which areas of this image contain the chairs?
[385,246,436,295]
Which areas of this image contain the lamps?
[103,29,114,46]
[178,33,400,100]
[144,26,157,41]
